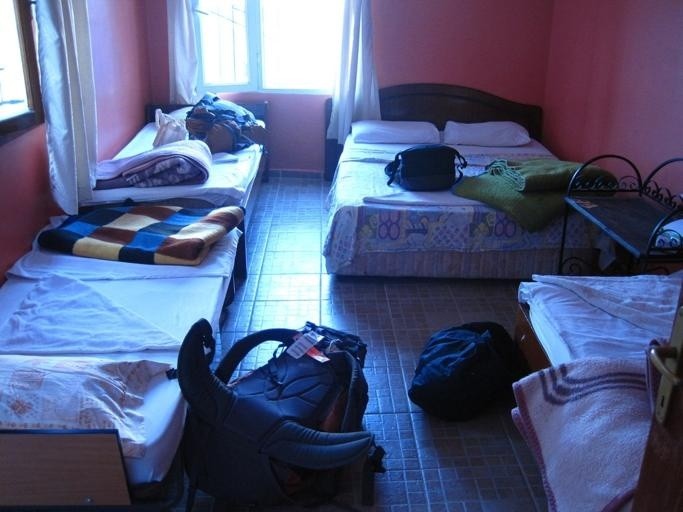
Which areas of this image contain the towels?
[510,338,670,512]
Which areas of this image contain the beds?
[325,84,618,285]
[0,207,242,512]
[514,271,683,374]
[80,100,270,279]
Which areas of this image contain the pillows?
[444,120,530,147]
[0,354,172,461]
[350,121,440,144]
[167,106,193,121]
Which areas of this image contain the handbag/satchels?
[385,144,465,190]
[408,320,514,423]
[187,92,257,153]
[153,109,187,149]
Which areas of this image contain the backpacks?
[163,318,386,512]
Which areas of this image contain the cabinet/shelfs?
[558,154,683,274]
